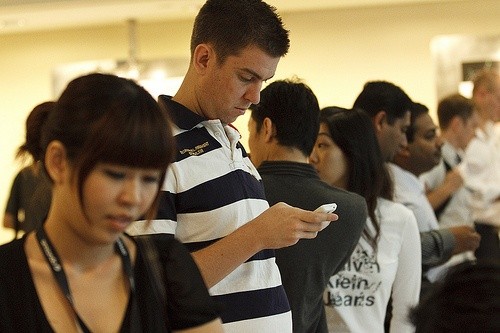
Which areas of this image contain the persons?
[4,102,54,234]
[248,78,368,333]
[0,72,223,333]
[461,68,500,227]
[309,106,421,333]
[418,93,476,228]
[124,0,338,333]
[384,103,452,286]
[352,80,480,301]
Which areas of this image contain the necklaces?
[37,226,135,333]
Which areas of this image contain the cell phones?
[313,203,337,213]
